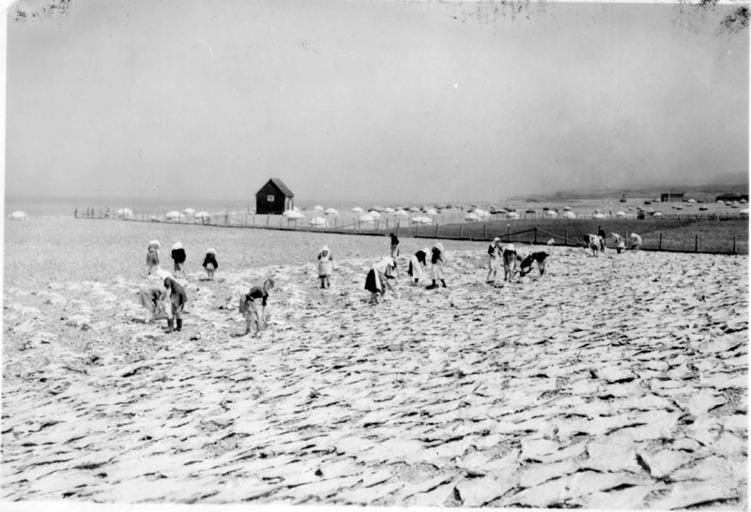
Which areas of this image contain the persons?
[145,238,160,277]
[486,236,548,286]
[636,210,645,221]
[171,241,186,272]
[584,224,643,257]
[202,247,218,279]
[239,279,275,336]
[317,248,333,290]
[364,230,446,308]
[161,276,187,334]
